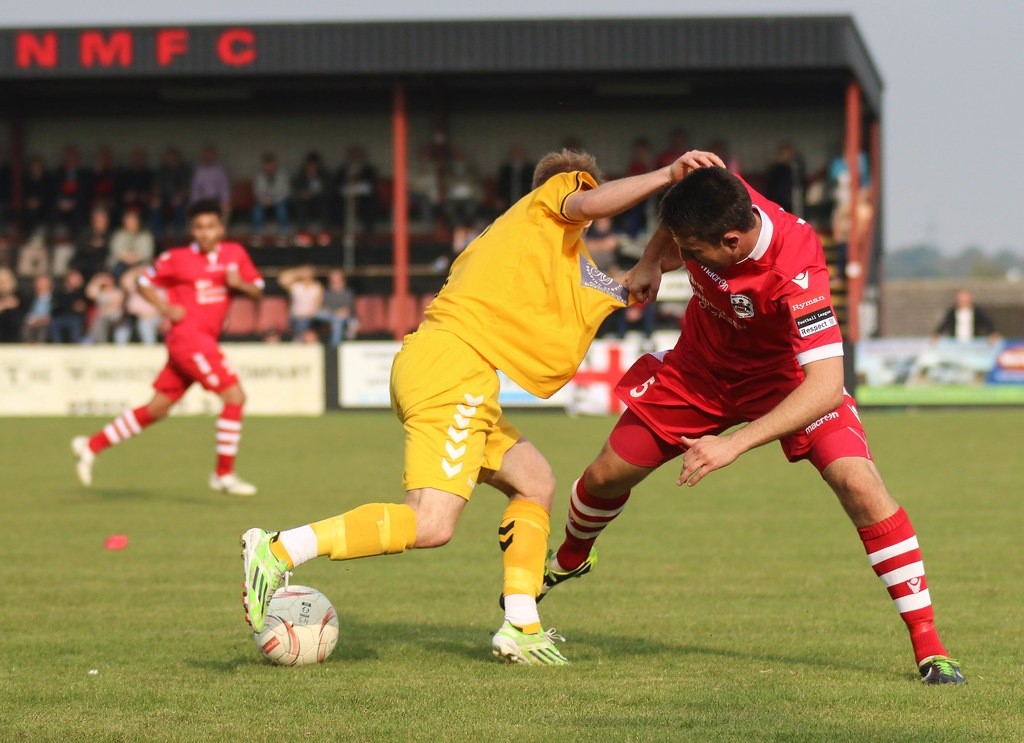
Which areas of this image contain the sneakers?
[242,526,293,634]
[72,435,95,487]
[491,620,570,666]
[209,469,258,495]
[918,655,967,686]
[499,543,598,609]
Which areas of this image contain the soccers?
[253,585,340,666]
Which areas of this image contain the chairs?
[83,293,438,337]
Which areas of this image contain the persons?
[72,200,265,497]
[499,166,963,684]
[242,149,727,668]
[938,290,996,338]
[0,136,878,348]
